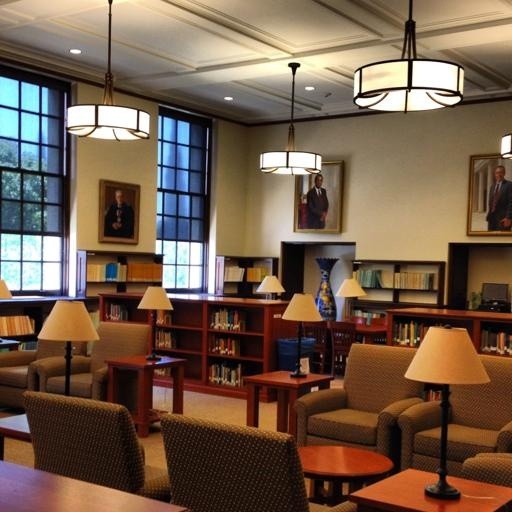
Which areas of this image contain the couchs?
[292,342,491,487]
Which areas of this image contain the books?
[153,314,180,378]
[480,329,512,357]
[222,265,268,283]
[350,309,428,347]
[108,304,130,320]
[208,308,250,388]
[87,262,163,282]
[0,315,37,351]
[351,269,434,291]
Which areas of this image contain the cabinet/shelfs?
[447,243,493,312]
[78,248,168,298]
[349,258,448,310]
[384,306,492,355]
[212,253,279,300]
[279,242,353,333]
[95,289,302,403]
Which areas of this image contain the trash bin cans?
[277,337,316,372]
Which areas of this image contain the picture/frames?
[99,179,141,245]
[467,152,511,235]
[293,160,345,234]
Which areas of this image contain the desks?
[1,299,91,351]
[0,459,189,512]
[1,415,33,463]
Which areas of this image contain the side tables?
[242,370,333,436]
[106,357,185,438]
[349,467,512,512]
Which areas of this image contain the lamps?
[500,133,511,158]
[333,275,368,304]
[258,63,322,176]
[403,324,492,500]
[0,279,13,300]
[66,0,151,142]
[351,0,465,114]
[254,274,287,299]
[281,289,325,379]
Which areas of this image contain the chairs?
[0,319,151,412]
[160,411,310,511]
[22,390,170,502]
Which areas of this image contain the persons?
[486,166,512,231]
[104,189,134,239]
[307,174,329,228]
